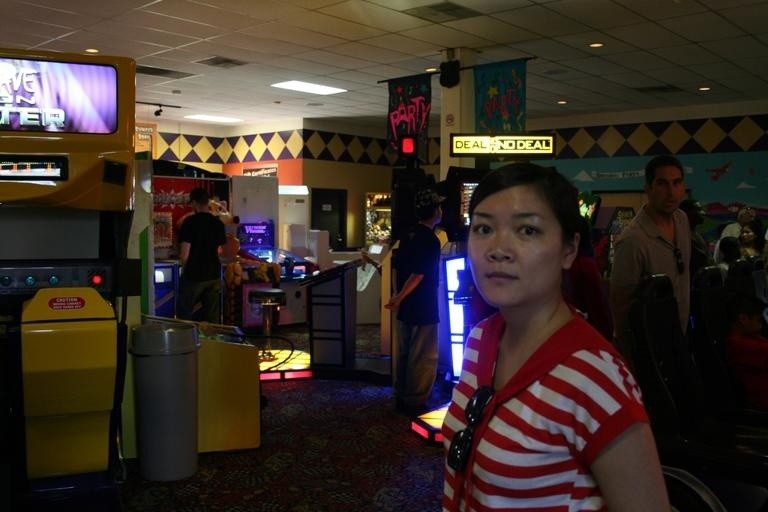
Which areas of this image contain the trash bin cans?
[129,322,200,482]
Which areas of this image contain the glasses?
[446,383,495,473]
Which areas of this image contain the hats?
[187,186,208,204]
[413,189,447,209]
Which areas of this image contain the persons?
[608,155,690,333]
[441,162,670,512]
[682,199,761,288]
[384,189,445,412]
[728,297,767,410]
[178,187,226,324]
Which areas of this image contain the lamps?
[136,101,181,116]
